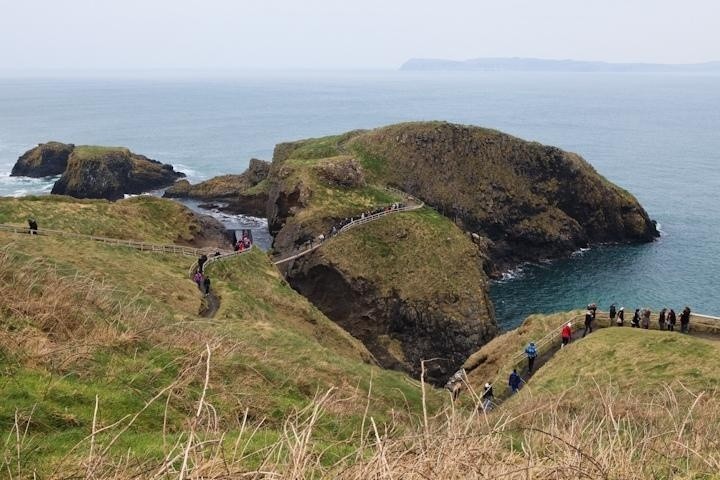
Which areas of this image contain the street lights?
[617,317,622,323]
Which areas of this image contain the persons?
[561,323,571,347]
[581,301,691,338]
[204,275,210,296]
[27,218,37,234]
[525,342,538,375]
[329,199,406,237]
[194,237,254,288]
[482,383,495,410]
[508,368,519,393]
